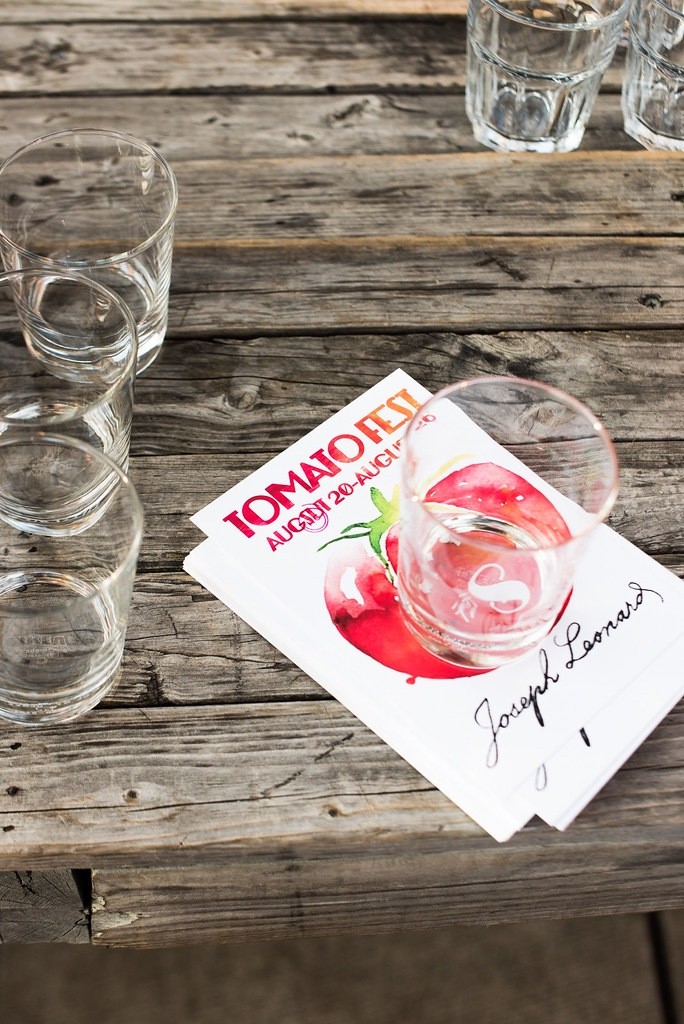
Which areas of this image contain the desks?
[0,0,684,946]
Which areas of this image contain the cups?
[0,127,179,388]
[0,265,139,539]
[0,431,146,729]
[397,376,621,671]
[466,0,634,155]
[621,0,684,151]
